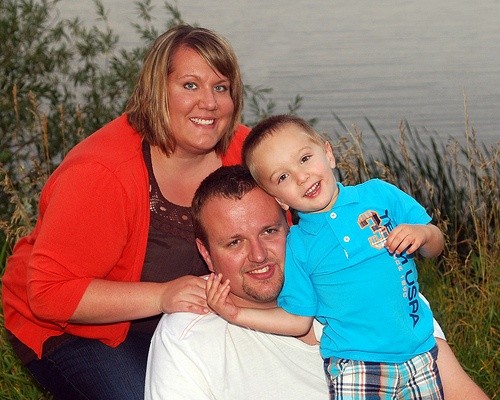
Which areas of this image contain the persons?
[204,114,447,400]
[144,162,493,400]
[0,23,299,399]
